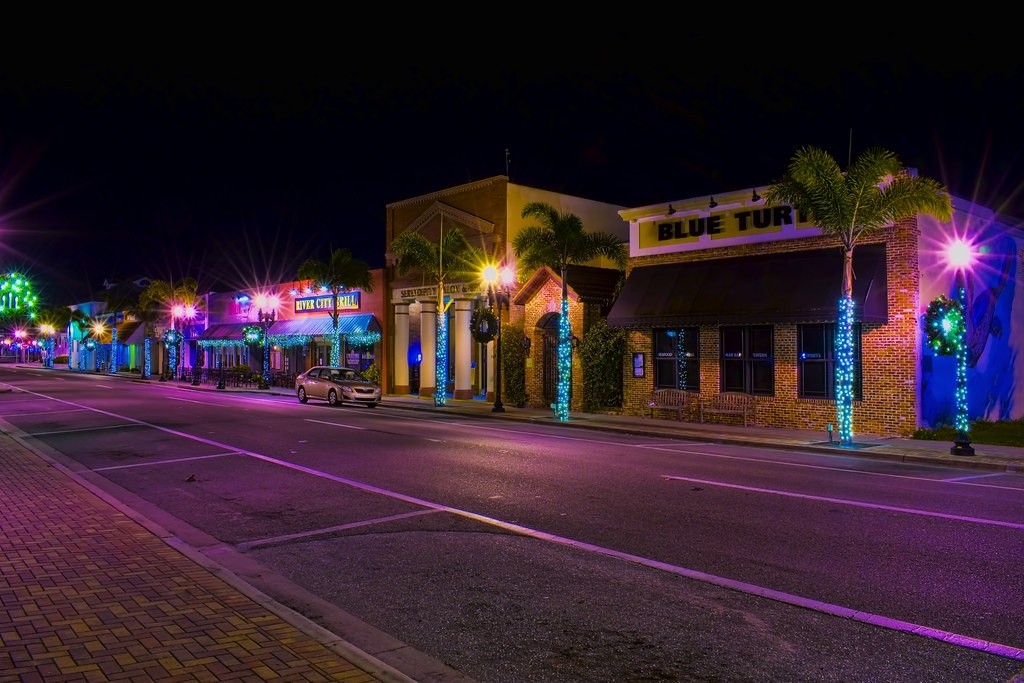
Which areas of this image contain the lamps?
[752,187,761,202]
[668,203,676,215]
[710,195,718,208]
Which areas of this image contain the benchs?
[699,392,754,427]
[647,388,693,420]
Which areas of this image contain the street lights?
[484,265,512,413]
[945,238,976,455]
[15,330,27,362]
[41,324,54,367]
[254,294,280,390]
[175,306,194,382]
[96,324,103,372]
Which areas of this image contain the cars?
[295,366,382,408]
